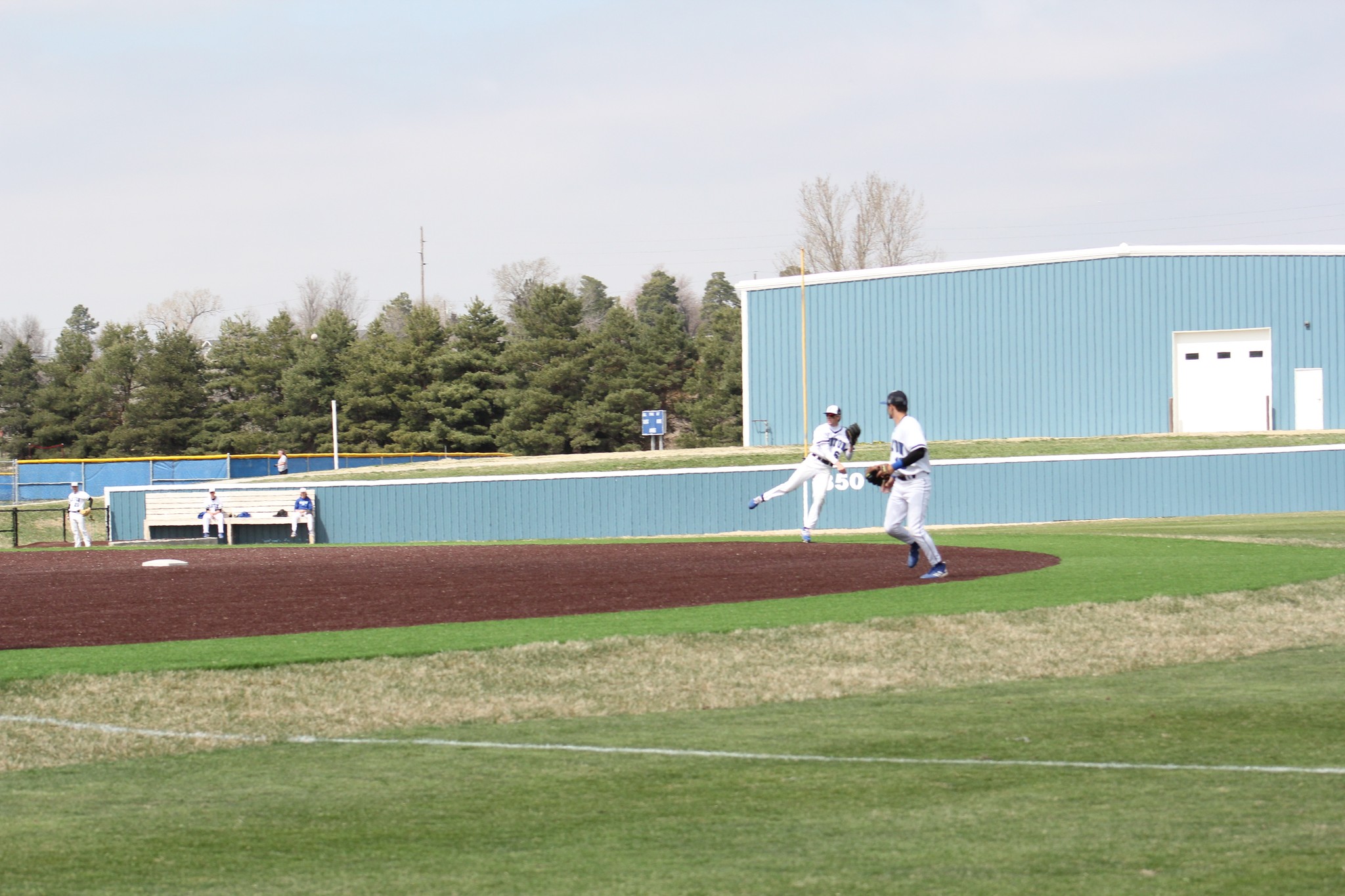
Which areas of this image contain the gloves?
[274,464,277,467]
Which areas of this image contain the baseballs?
[310,334,318,341]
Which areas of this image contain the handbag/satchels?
[273,509,287,517]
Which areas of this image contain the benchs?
[143,490,316,545]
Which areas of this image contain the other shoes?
[203,534,210,537]
[308,531,314,536]
[291,532,297,537]
[218,534,224,538]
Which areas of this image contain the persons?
[864,389,949,579]
[748,405,861,543]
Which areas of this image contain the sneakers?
[919,563,948,578]
[908,543,920,567]
[801,527,811,541]
[748,494,765,509]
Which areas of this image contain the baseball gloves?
[865,464,891,487]
[844,423,861,445]
[79,507,91,517]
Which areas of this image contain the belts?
[72,511,80,512]
[897,475,915,481]
[812,452,833,466]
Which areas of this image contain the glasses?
[826,415,835,417]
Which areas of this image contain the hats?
[209,487,215,491]
[300,488,306,492]
[879,390,907,404]
[823,405,841,414]
[71,482,78,486]
